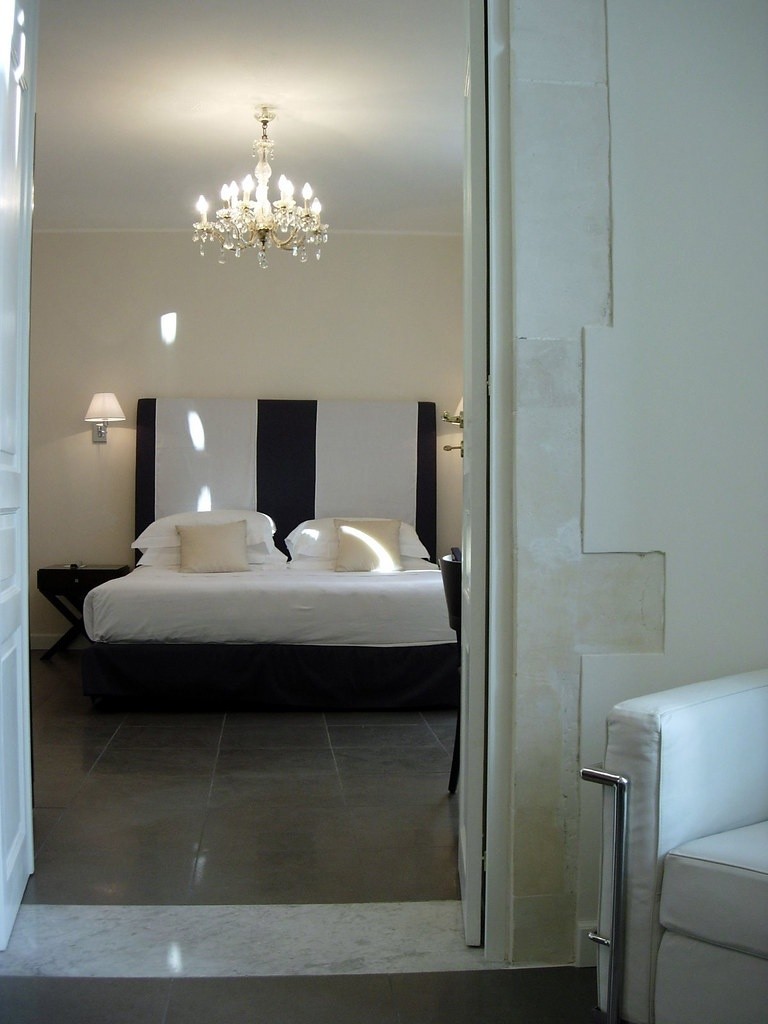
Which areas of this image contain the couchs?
[577,667,768,1023]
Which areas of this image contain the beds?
[81,395,458,706]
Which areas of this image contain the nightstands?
[36,564,132,662]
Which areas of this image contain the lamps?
[84,393,126,441]
[441,396,464,455]
[190,106,329,270]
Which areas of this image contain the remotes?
[451,548,461,561]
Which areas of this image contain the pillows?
[136,544,287,568]
[337,518,403,571]
[179,520,249,573]
[284,517,430,561]
[132,509,277,552]
